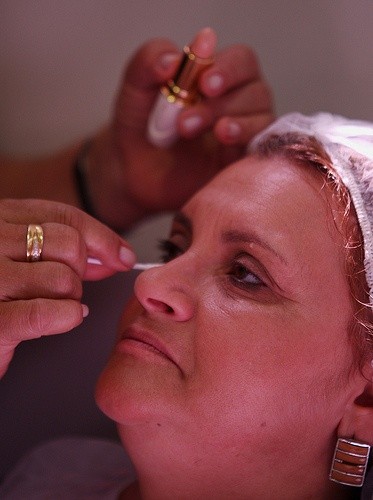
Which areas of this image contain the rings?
[25,224,44,263]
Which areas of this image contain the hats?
[249,110,373,312]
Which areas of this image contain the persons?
[1,38,279,382]
[0,109,373,500]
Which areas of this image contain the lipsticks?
[144,25,218,151]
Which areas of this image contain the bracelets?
[70,137,130,239]
